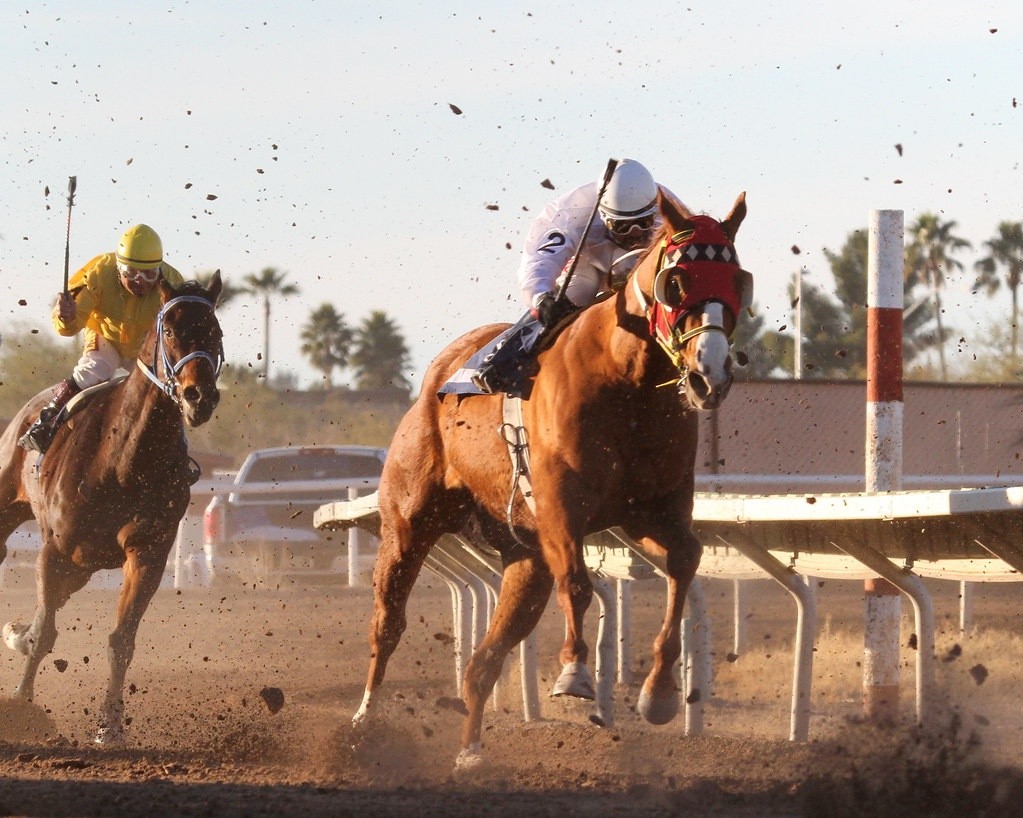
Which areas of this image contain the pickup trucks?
[202,443,388,584]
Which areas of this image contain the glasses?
[120,265,159,281]
[605,213,655,235]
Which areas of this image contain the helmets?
[597,158,657,245]
[117,224,162,270]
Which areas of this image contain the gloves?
[531,291,573,328]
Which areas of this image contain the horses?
[0,267,225,752]
[349,187,753,777]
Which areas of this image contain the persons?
[472,158,694,389]
[18,226,201,486]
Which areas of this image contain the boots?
[472,286,579,391]
[18,376,83,453]
